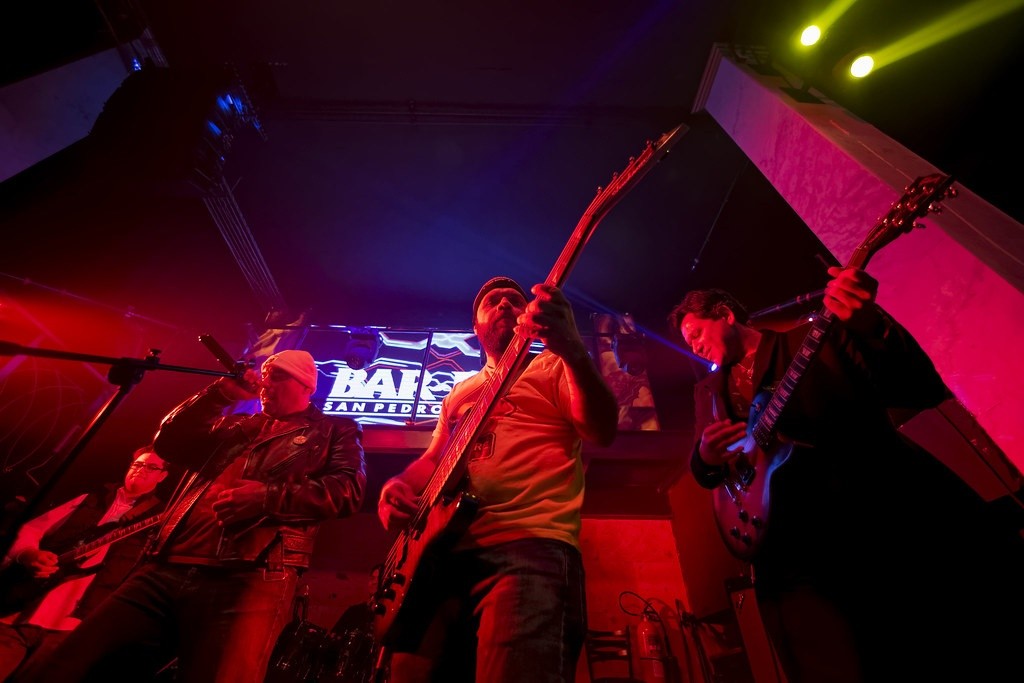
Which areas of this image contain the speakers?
[724,576,789,683]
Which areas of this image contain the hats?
[473,277,528,319]
[261,350,318,395]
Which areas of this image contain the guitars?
[370,115,698,655]
[0,509,171,620]
[708,171,963,564]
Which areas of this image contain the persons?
[0,350,367,683]
[377,276,618,683]
[669,266,1024,683]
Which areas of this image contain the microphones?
[197,333,260,394]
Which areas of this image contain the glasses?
[130,462,163,472]
